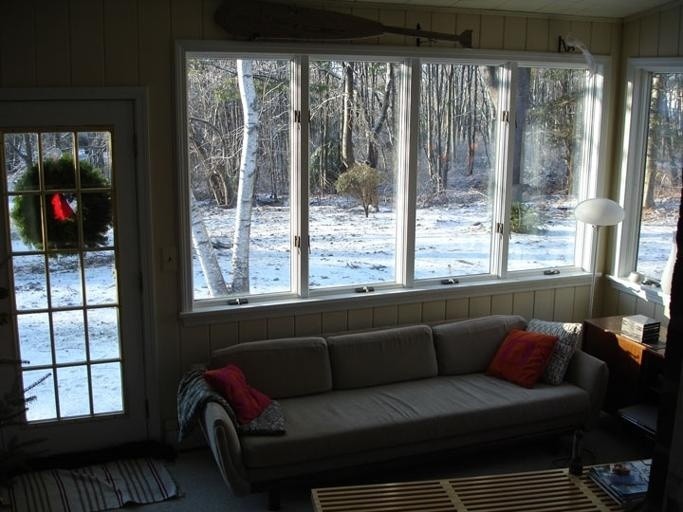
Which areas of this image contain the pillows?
[523,318,582,386]
[483,328,559,389]
[204,363,273,426]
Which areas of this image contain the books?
[620,313,660,343]
[587,459,654,506]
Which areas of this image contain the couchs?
[203,313,612,507]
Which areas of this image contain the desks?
[583,313,672,456]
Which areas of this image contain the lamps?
[573,196,628,319]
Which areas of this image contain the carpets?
[3,453,190,511]
